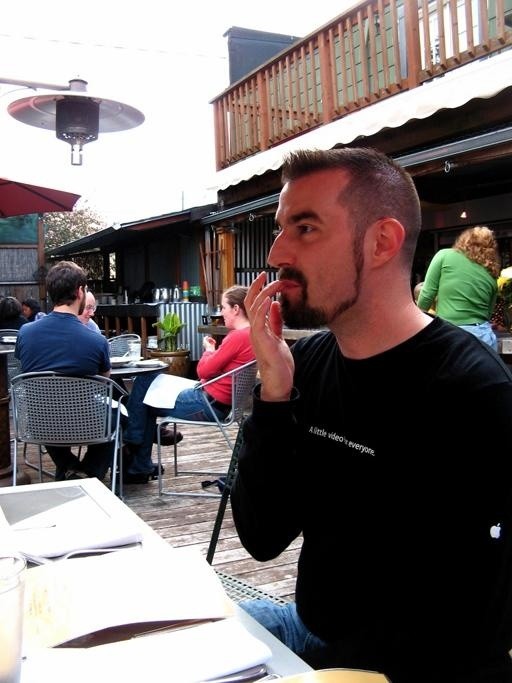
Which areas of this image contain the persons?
[415,220,500,356]
[229,147,511,681]
[79,288,182,449]
[1,294,44,337]
[15,261,119,485]
[107,284,260,487]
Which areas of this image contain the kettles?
[152,288,180,302]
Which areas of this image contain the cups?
[97,296,116,305]
[148,336,158,348]
[0,552,28,683]
[127,340,141,368]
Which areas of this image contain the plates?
[2,336,17,342]
[110,357,130,368]
[136,360,161,368]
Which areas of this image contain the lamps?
[0,74,144,166]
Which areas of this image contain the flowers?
[497,265,512,334]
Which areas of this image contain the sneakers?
[111,462,164,484]
[153,424,183,446]
[65,470,88,479]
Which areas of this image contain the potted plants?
[150,313,191,378]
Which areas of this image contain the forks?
[19,548,122,566]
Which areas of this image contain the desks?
[1,477,315,682]
[93,303,158,357]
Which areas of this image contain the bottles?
[182,281,189,302]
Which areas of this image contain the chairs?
[205,416,300,607]
